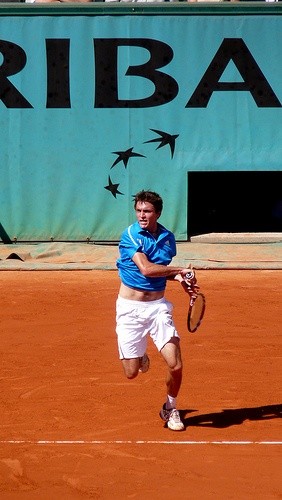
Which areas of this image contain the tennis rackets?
[184,272,206,334]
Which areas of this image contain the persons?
[115,190,200,431]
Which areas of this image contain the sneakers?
[138,354,150,372]
[159,402,185,431]
